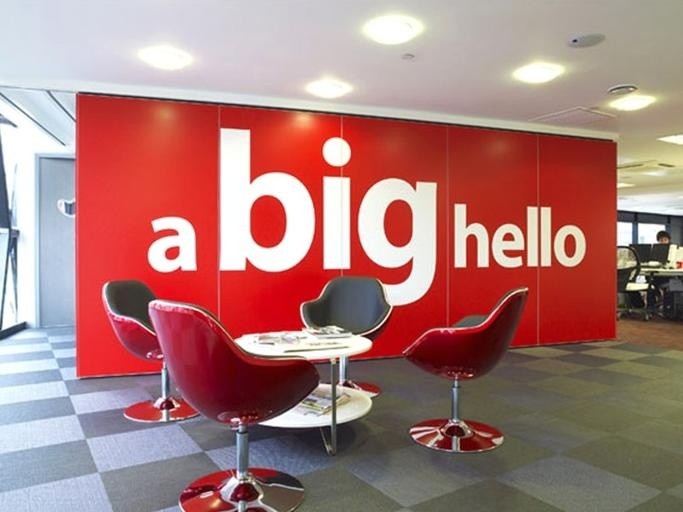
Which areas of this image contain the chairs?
[148,301,319,510]
[301,276,395,399]
[102,278,200,424]
[402,287,528,452]
[616,246,660,321]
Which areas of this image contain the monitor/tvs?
[628,243,651,262]
[651,242,670,262]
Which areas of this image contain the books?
[252,320,353,418]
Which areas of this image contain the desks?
[638,270,682,321]
[236,330,373,457]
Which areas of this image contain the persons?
[643,229,676,317]
